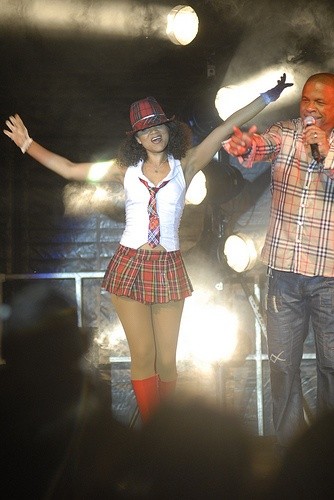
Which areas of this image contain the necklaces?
[145,159,169,174]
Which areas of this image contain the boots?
[131,375,161,428]
[159,376,178,401]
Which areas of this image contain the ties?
[139,177,170,248]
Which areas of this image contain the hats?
[124,95,176,136]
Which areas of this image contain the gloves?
[261,73,294,105]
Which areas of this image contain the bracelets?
[20,138,33,155]
[259,89,272,106]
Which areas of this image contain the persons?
[220,73,334,437]
[2,72,294,427]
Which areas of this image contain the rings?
[313,131,318,139]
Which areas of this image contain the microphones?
[304,116,320,160]
